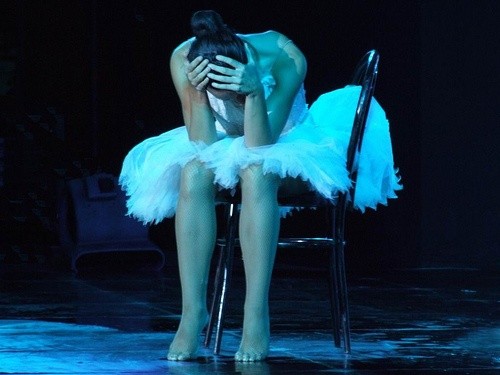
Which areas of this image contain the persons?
[167,10,307,362]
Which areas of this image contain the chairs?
[205,49,380,354]
